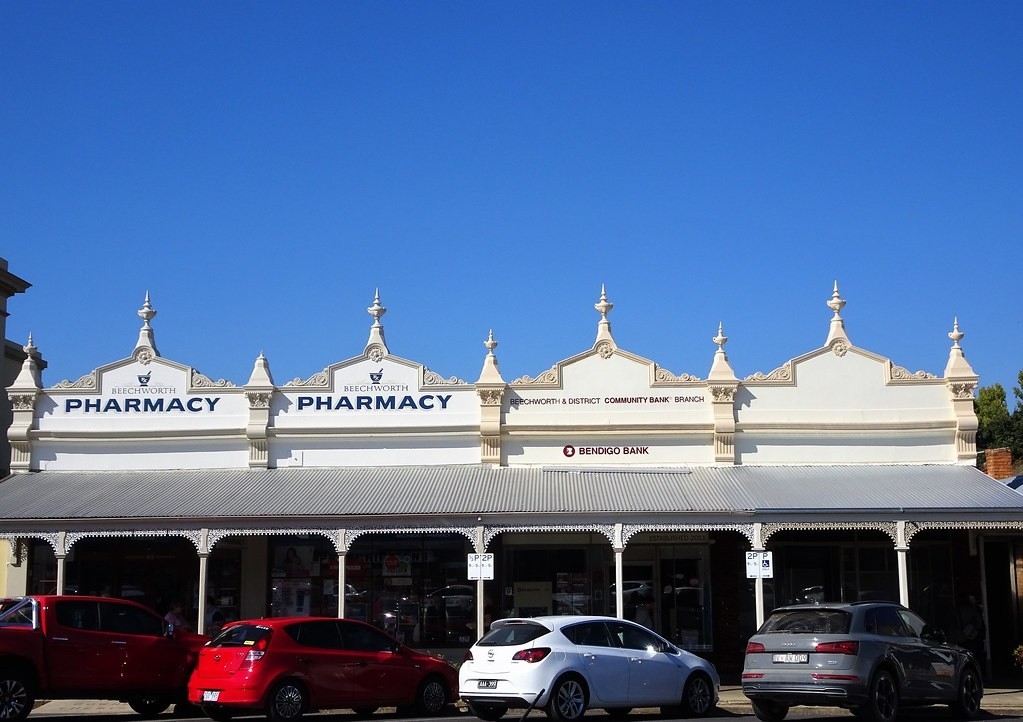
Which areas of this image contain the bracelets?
[210,626,213,631]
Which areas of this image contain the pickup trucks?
[0,592,213,722]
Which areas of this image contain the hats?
[207,595,215,605]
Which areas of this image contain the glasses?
[177,605,184,608]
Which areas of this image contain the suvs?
[740,598,986,722]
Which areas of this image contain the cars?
[420,583,476,612]
[609,579,655,606]
[185,616,461,722]
[457,613,720,722]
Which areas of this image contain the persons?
[466,593,491,645]
[636,596,655,630]
[164,602,192,630]
[200,595,225,635]
